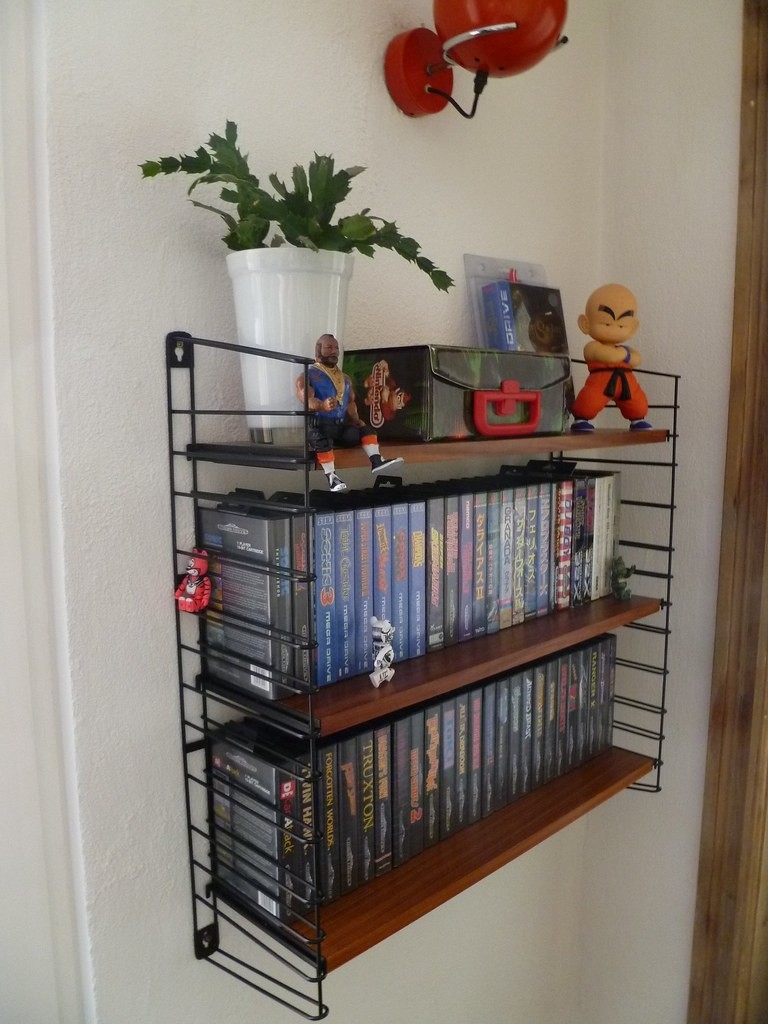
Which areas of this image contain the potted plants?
[137,118,459,444]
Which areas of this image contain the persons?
[573,284,652,433]
[298,333,404,492]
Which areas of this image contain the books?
[195,466,623,695]
[204,631,614,919]
[482,280,576,421]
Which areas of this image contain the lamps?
[385,0,569,121]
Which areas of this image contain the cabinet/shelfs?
[164,330,682,1020]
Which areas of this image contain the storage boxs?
[341,346,572,451]
